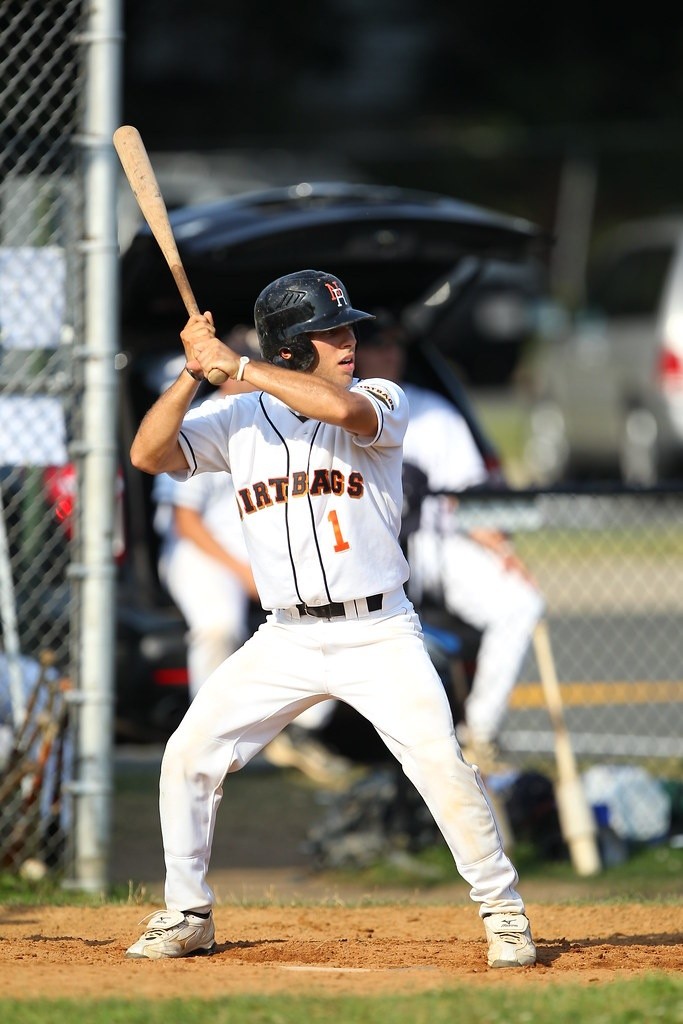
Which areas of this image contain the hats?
[355,309,408,347]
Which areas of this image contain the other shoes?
[16,858,51,881]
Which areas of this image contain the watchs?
[237,356,249,382]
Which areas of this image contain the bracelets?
[185,363,205,382]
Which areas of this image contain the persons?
[339,308,544,793]
[125,271,538,970]
[160,325,281,779]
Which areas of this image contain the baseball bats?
[530,613,603,879]
[112,123,231,387]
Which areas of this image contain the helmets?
[253,269,376,371]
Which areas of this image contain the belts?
[273,592,383,619]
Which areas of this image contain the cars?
[554,223,683,497]
[0,186,533,762]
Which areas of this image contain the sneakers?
[483,911,536,969]
[457,726,511,776]
[267,732,350,786]
[124,910,217,960]
